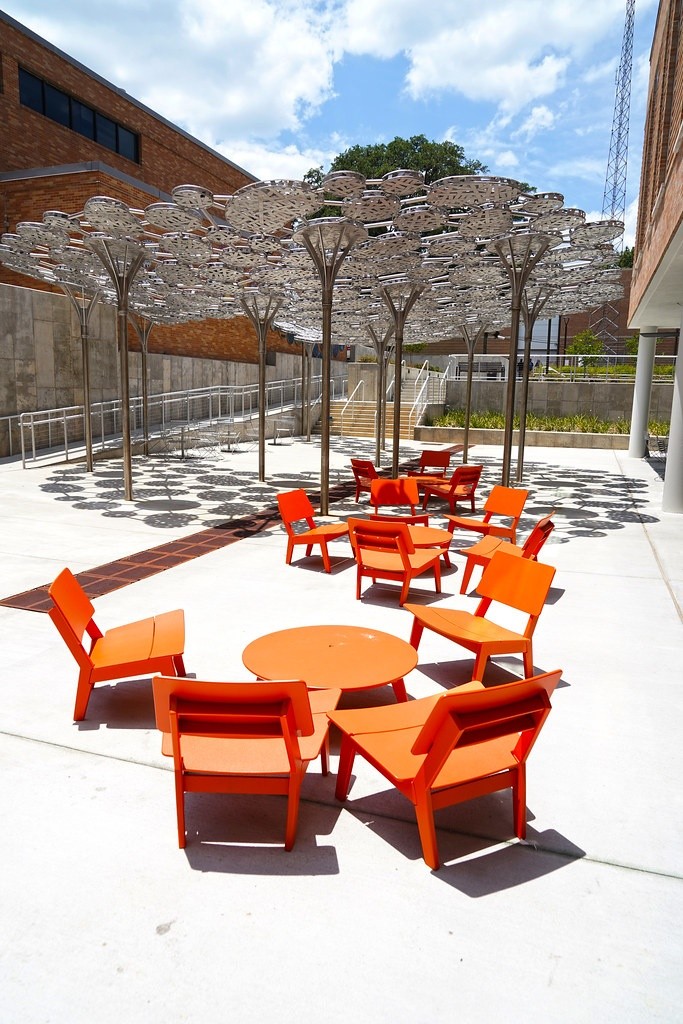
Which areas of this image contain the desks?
[398,477,450,499]
[171,426,199,459]
[265,419,288,445]
[242,624,419,706]
[403,525,453,568]
[217,423,240,452]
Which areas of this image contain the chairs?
[459,512,556,594]
[150,675,343,851]
[368,479,429,527]
[657,440,668,460]
[407,451,450,479]
[347,518,448,608]
[325,669,565,872]
[403,551,553,684]
[48,568,185,719]
[278,489,356,573]
[422,465,482,515]
[148,416,297,462]
[443,486,527,563]
[351,459,393,503]
